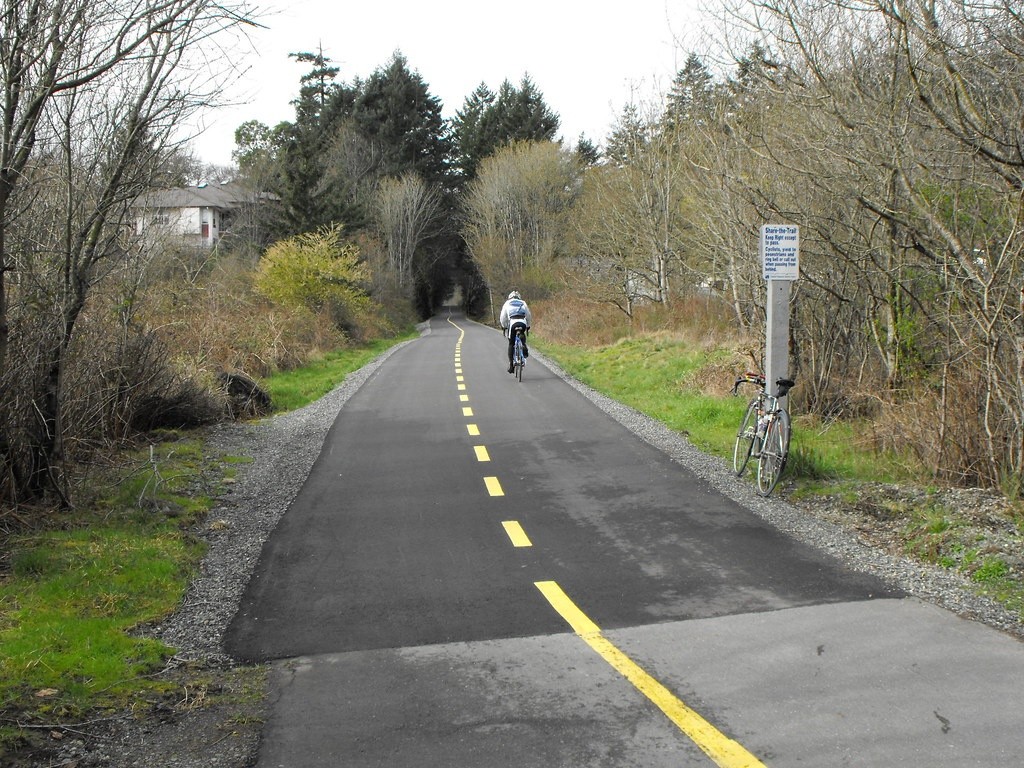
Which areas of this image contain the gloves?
[502,327,507,329]
[526,326,530,330]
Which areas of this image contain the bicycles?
[730,370,795,497]
[501,324,530,382]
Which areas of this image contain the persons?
[500,291,531,374]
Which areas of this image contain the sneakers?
[523,346,528,357]
[508,367,514,373]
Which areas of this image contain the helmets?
[508,290,521,300]
